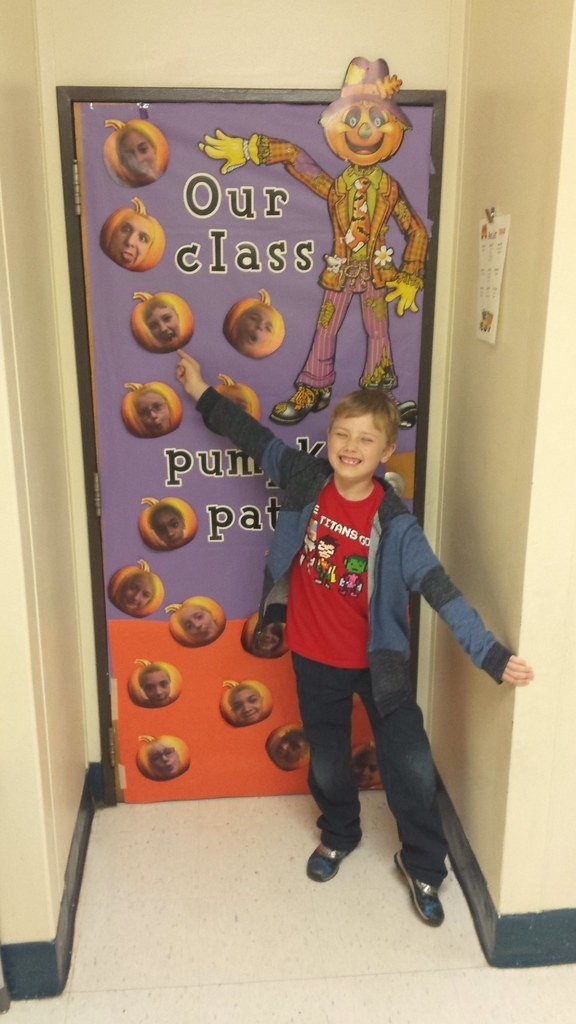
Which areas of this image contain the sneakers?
[394,849,445,926]
[307,842,348,882]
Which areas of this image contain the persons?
[135,389,171,434]
[219,388,249,411]
[119,572,156,611]
[117,127,156,178]
[350,749,381,788]
[147,742,180,774]
[254,622,282,653]
[276,729,309,764]
[227,685,263,724]
[139,664,171,703]
[174,348,533,926]
[109,215,153,268]
[144,297,181,347]
[177,602,215,642]
[235,304,276,350]
[149,503,185,546]
